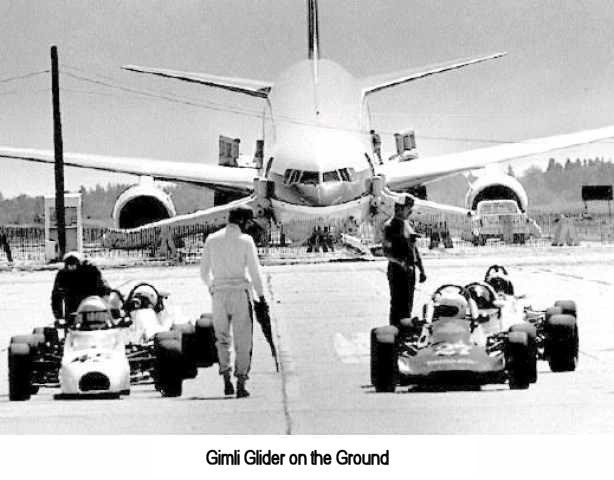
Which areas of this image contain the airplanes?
[0,1,613,250]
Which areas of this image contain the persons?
[200,204,265,398]
[385,192,426,325]
[51,252,104,319]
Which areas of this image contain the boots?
[235,374,249,398]
[222,371,235,395]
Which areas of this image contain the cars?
[7,283,216,397]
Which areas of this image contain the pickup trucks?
[467,200,528,245]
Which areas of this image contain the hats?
[228,204,258,223]
[395,195,415,207]
